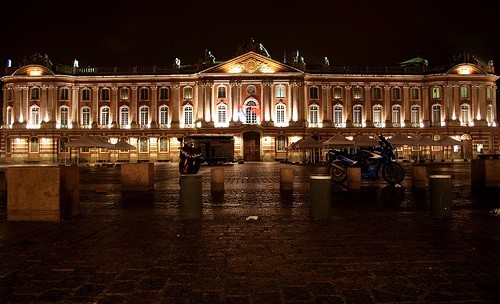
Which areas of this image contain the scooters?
[176,137,206,186]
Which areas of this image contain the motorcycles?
[326,131,406,185]
